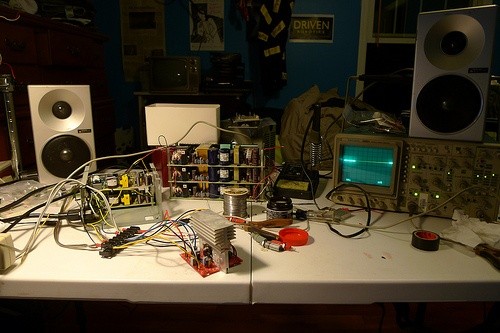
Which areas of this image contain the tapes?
[412,230,441,251]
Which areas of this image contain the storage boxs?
[143,101,223,146]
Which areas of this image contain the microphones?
[311,102,322,167]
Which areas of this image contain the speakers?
[27,84,97,183]
[408,5,496,141]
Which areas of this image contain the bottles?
[187,56,202,94]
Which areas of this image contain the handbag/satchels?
[279,82,382,169]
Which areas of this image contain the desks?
[0,175,500,333]
[134,80,253,151]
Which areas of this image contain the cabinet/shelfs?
[0,4,114,168]
